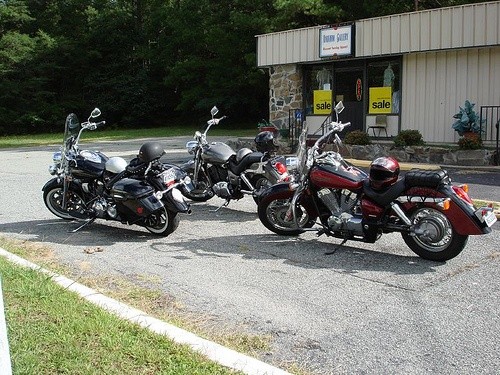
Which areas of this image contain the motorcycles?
[42,108,192,237]
[179,106,279,205]
[257,101,497,262]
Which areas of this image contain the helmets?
[235,147,253,162]
[254,131,279,152]
[138,142,167,164]
[105,156,129,174]
[369,157,400,193]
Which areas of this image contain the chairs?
[367,115,389,140]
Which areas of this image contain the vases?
[280,130,289,137]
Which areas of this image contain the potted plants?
[451,100,487,141]
[258,121,276,133]
